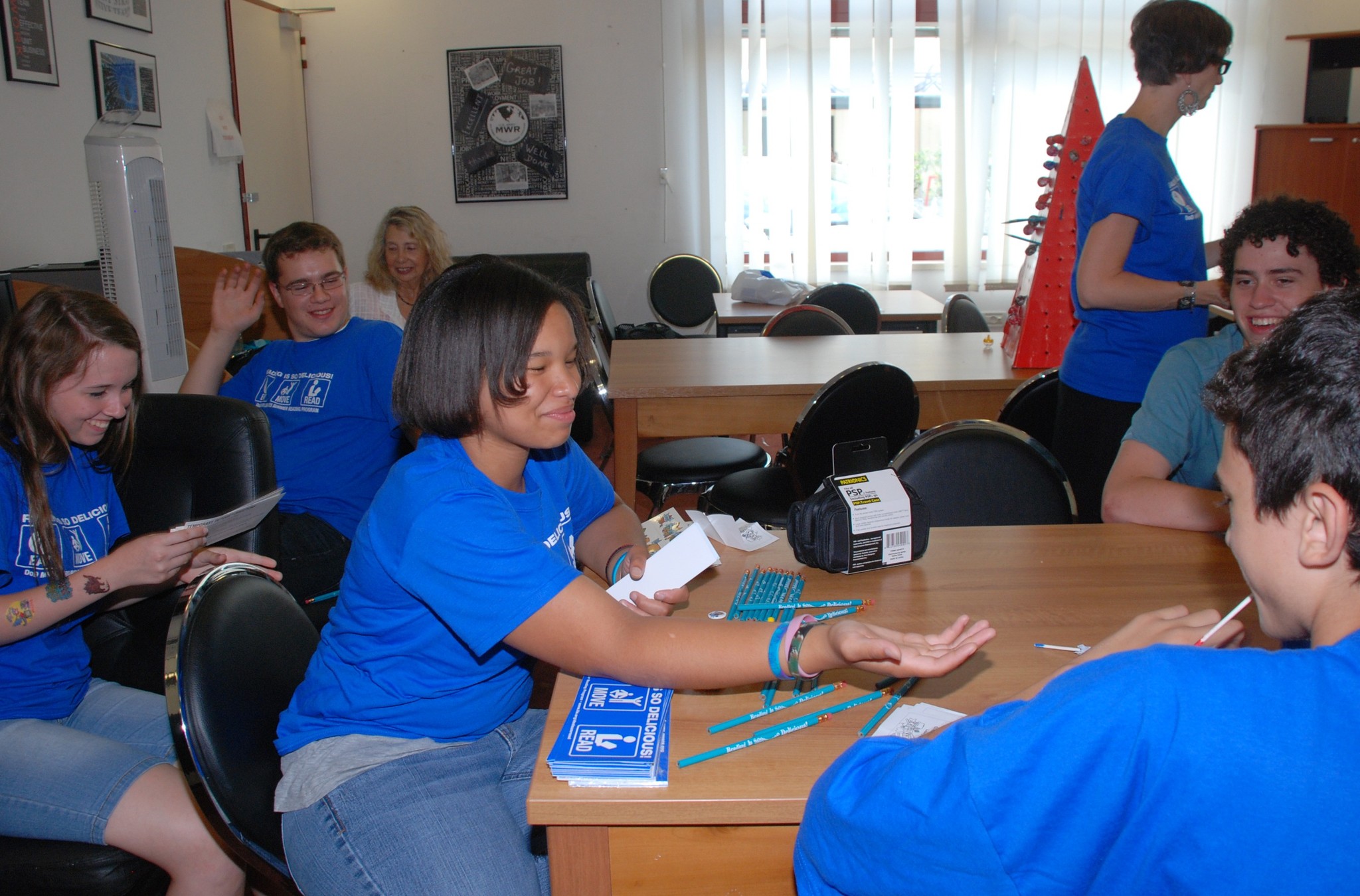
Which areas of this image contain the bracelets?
[605,543,633,587]
[768,614,821,679]
[1177,280,1194,310]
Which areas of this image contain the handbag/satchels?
[787,481,929,573]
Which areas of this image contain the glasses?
[270,271,346,296]
[1212,57,1231,76]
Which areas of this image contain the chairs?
[86,386,279,682]
[695,364,919,525]
[942,293,988,333]
[645,255,725,342]
[992,361,1060,451]
[889,416,1082,525]
[171,546,306,896]
[759,301,853,337]
[567,292,771,518]
[797,282,883,335]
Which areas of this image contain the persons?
[1052,0,1232,524]
[794,289,1360,896]
[1102,197,1360,532]
[273,261,996,896]
[348,206,453,332]
[178,221,404,598]
[0,287,282,896]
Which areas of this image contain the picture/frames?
[0,0,60,87]
[91,39,163,134]
[86,0,153,34]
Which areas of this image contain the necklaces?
[397,291,414,306]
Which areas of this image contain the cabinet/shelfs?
[1250,124,1360,248]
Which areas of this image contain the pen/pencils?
[811,679,818,690]
[753,687,894,736]
[763,680,778,707]
[792,679,802,698]
[676,712,831,768]
[788,605,865,622]
[706,678,847,734]
[738,598,875,610]
[875,677,898,689]
[305,590,340,605]
[759,683,771,701]
[857,677,918,738]
[726,564,805,622]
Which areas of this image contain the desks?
[519,506,1279,896]
[711,285,947,333]
[605,327,1044,512]
